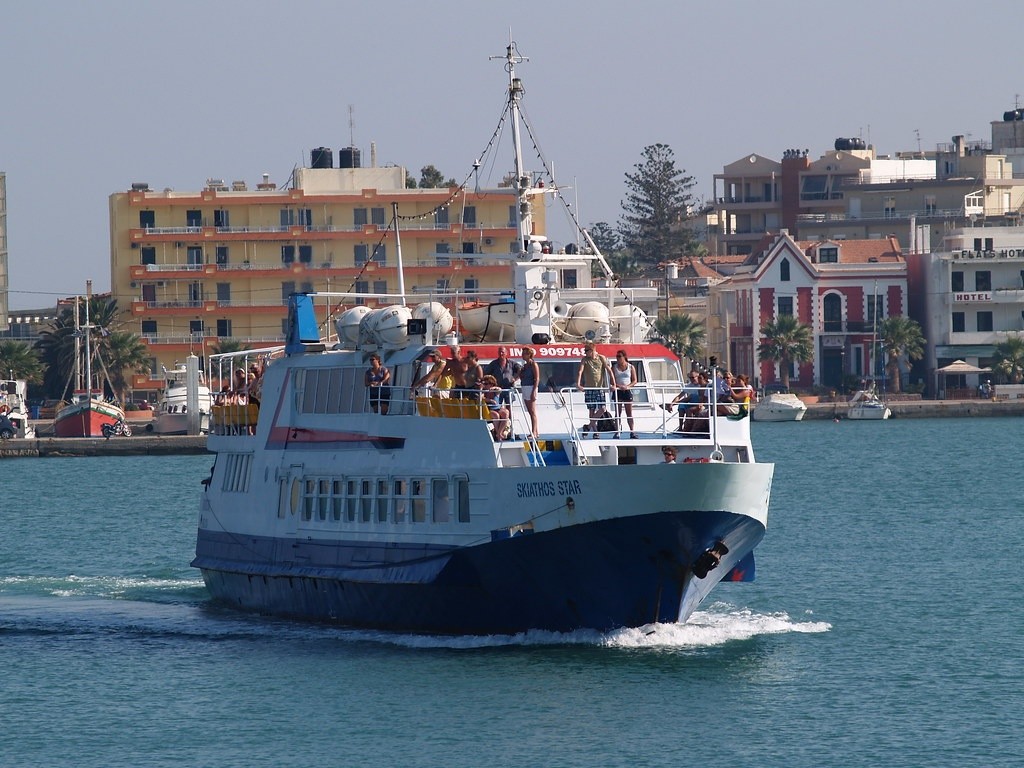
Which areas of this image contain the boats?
[193,21,776,647]
[0,368,36,438]
[753,393,808,422]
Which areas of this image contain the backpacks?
[598,409,617,431]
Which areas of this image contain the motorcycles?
[100,412,132,438]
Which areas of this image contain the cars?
[0,415,18,440]
[40,398,61,418]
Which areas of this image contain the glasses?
[482,382,491,385]
[688,376,697,379]
[369,358,376,361]
[722,376,730,380]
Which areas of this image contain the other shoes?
[495,435,504,442]
[582,424,590,439]
[673,429,683,434]
[681,434,696,438]
[630,433,639,439]
[524,434,540,439]
[613,432,620,438]
[593,433,599,439]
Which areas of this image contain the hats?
[235,368,244,373]
[239,393,245,397]
[249,363,259,369]
[428,350,443,358]
[248,373,256,380]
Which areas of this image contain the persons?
[578,343,615,440]
[658,447,677,464]
[411,346,523,441]
[610,351,639,440]
[668,369,753,439]
[519,346,540,439]
[216,363,265,436]
[365,354,390,415]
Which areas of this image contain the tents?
[936,359,996,401]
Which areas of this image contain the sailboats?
[152,278,220,432]
[849,286,897,422]
[50,299,130,439]
